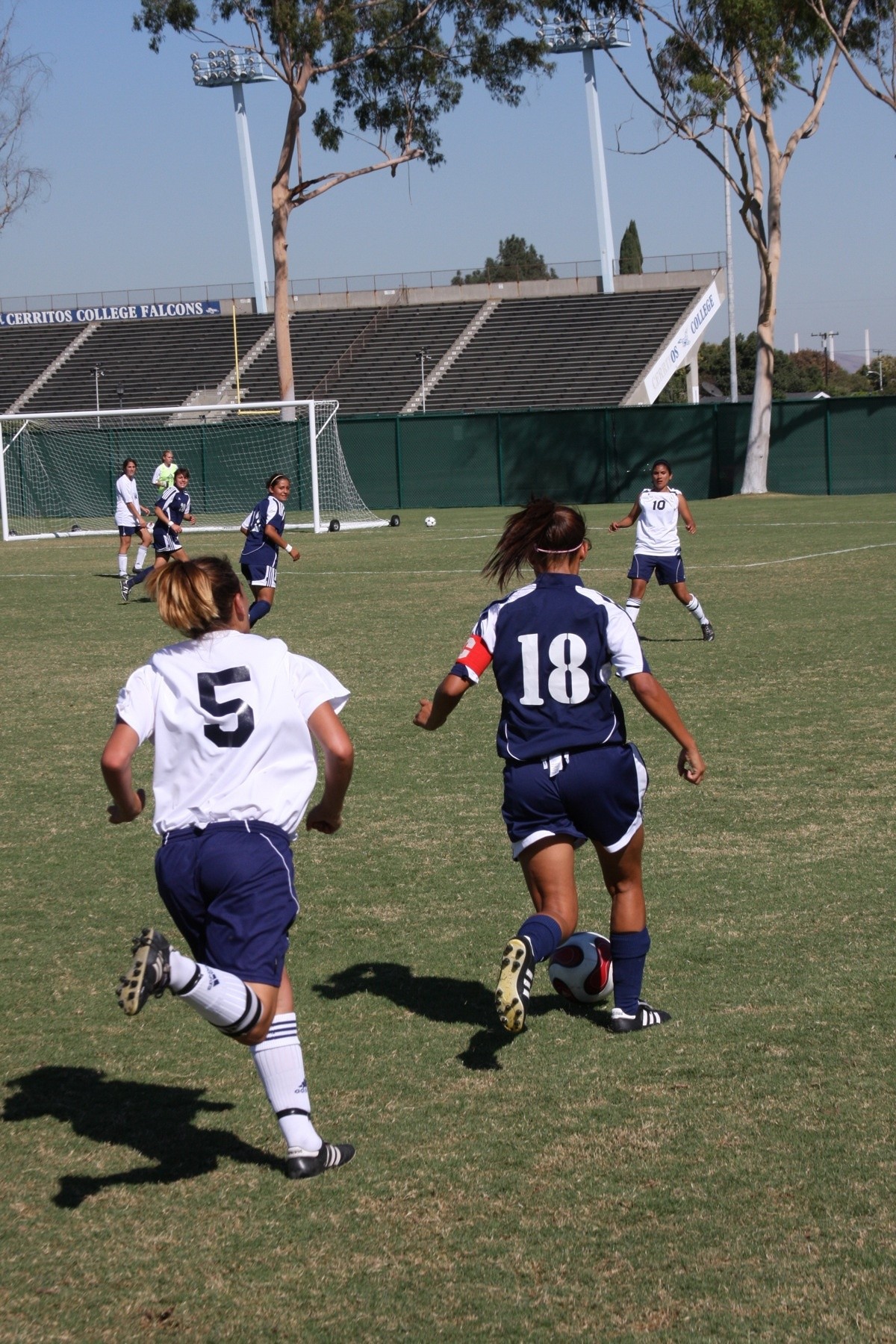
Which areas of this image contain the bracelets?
[168,520,174,528]
[285,543,293,553]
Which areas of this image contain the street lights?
[535,12,632,294]
[190,47,279,314]
[415,346,432,412]
[90,362,107,429]
[114,381,125,428]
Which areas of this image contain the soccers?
[425,516,436,527]
[547,931,614,1000]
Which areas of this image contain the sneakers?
[700,621,714,641]
[608,999,671,1034]
[285,1140,357,1180]
[495,935,535,1032]
[117,573,135,579]
[120,580,131,602]
[132,566,143,574]
[115,926,171,1016]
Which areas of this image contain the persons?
[100,554,357,1180]
[119,467,196,602]
[609,459,716,642]
[412,493,707,1035]
[239,473,301,629]
[114,459,152,580]
[152,450,178,499]
